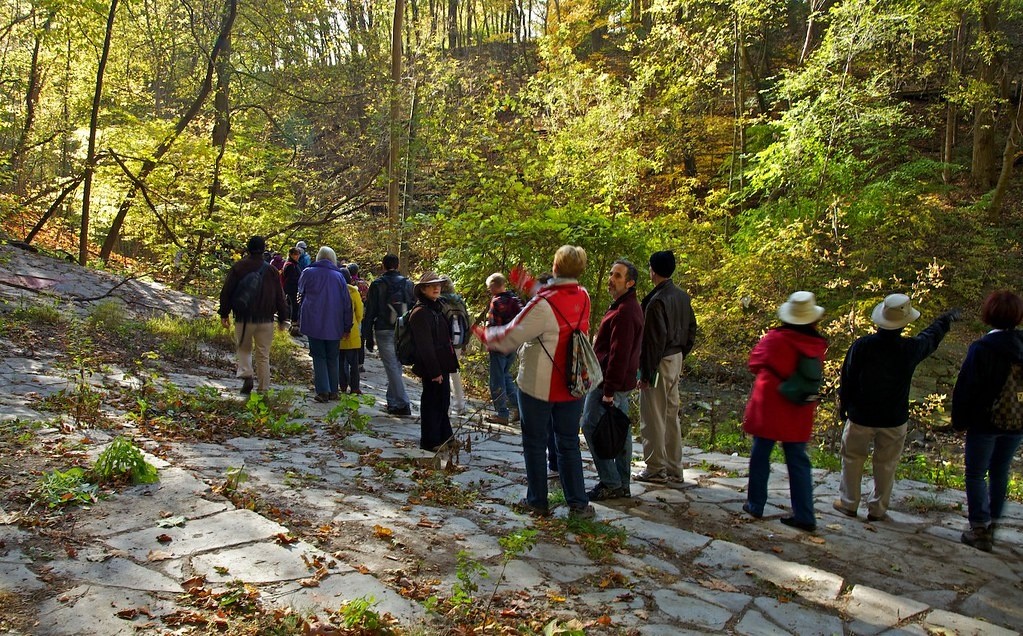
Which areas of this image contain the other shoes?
[240,378,253,394]
[351,389,361,394]
[780,516,816,532]
[288,323,303,336]
[341,385,347,393]
[743,504,762,519]
[435,438,462,452]
[315,393,338,404]
[387,406,411,415]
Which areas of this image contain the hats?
[650,251,676,278]
[778,291,824,325]
[296,241,307,250]
[413,272,446,298]
[873,293,920,330]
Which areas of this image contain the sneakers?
[632,468,668,482]
[668,475,683,483]
[586,482,631,500]
[867,513,891,522]
[961,525,995,553]
[520,497,549,517]
[832,498,857,517]
[569,506,595,521]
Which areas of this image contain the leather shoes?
[486,414,508,425]
[510,408,519,421]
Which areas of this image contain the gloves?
[946,307,961,321]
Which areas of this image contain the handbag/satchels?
[590,406,630,461]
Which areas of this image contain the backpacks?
[438,297,470,348]
[394,299,438,367]
[989,364,1023,431]
[231,261,269,315]
[765,350,821,406]
[535,295,603,399]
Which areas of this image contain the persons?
[438,275,472,417]
[262,241,371,372]
[217,236,288,396]
[630,249,697,483]
[482,272,521,426]
[338,268,364,396]
[473,245,595,520]
[365,252,415,416]
[407,272,461,452]
[296,247,354,404]
[580,260,645,501]
[952,288,1023,552]
[742,291,829,529]
[831,293,960,518]
